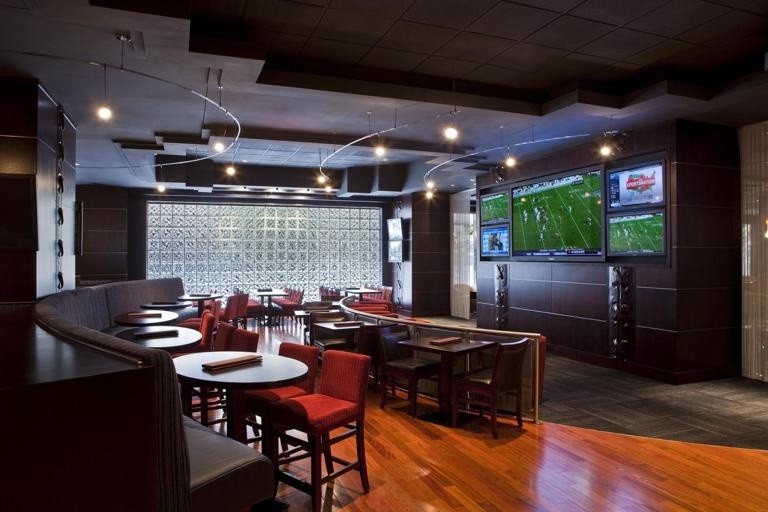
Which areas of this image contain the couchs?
[453,283,477,313]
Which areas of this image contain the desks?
[116,325,203,357]
[396,336,495,426]
[115,310,179,326]
[173,350,309,441]
[141,302,192,310]
[313,321,378,352]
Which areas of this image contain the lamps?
[97,64,237,193]
[318,115,611,198]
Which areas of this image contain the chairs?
[356,323,398,395]
[176,283,396,353]
[271,350,372,512]
[245,342,319,457]
[452,338,530,439]
[309,312,345,361]
[377,325,443,418]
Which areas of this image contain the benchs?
[36,285,279,512]
[103,277,199,333]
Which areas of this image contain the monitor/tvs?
[479,225,510,257]
[510,164,605,262]
[606,208,665,258]
[386,218,404,240]
[606,160,668,212]
[480,190,510,224]
[387,240,404,263]
